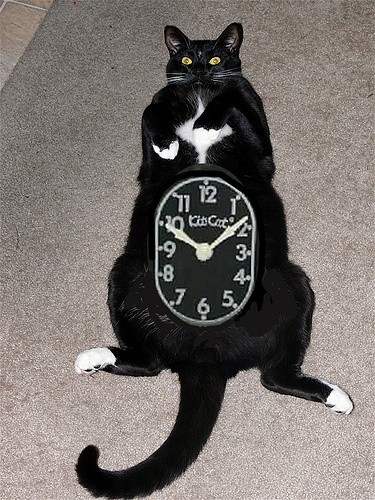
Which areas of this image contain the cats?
[74,22,354,500]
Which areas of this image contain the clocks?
[149,165,263,330]
